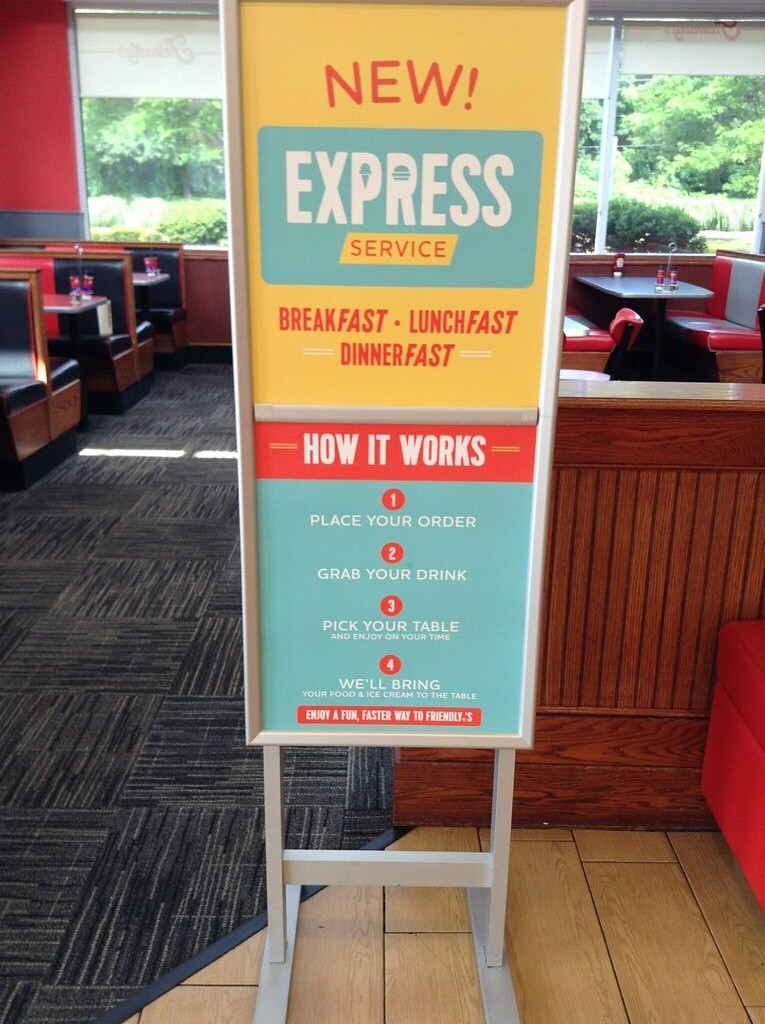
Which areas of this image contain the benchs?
[700,619,765,914]
[2,234,765,493]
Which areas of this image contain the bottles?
[82,269,95,297]
[152,254,159,271]
[657,265,666,285]
[669,266,677,284]
[68,270,81,297]
[143,253,154,277]
